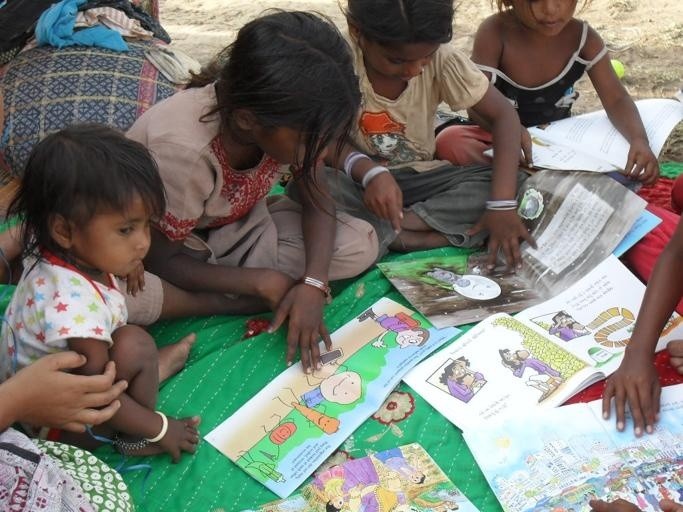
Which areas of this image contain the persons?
[281,0,538,276]
[120,6,379,375]
[603,171,683,437]
[0,122,199,463]
[0,350,129,432]
[436,1,661,186]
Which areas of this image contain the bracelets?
[300,275,328,292]
[484,200,518,210]
[342,150,371,179]
[144,410,168,442]
[359,165,389,189]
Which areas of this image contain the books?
[244,441,480,512]
[460,381,682,511]
[401,253,682,433]
[202,296,463,498]
[482,98,682,176]
[376,169,663,329]
